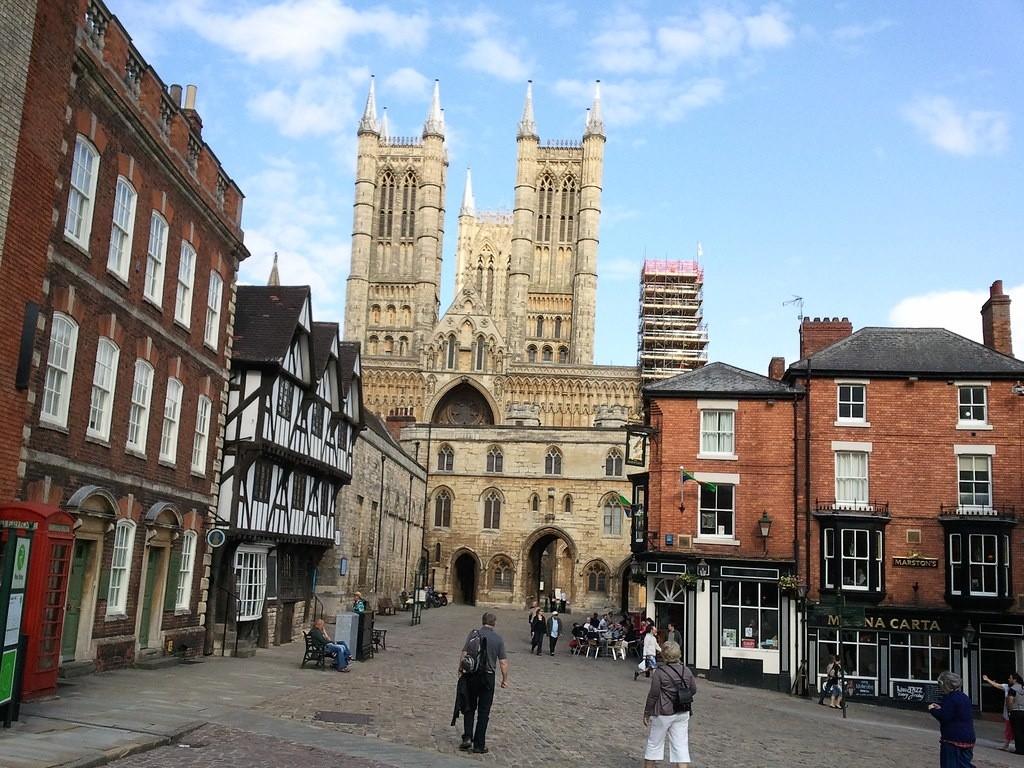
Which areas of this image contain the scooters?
[422,585,448,610]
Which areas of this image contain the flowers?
[679,573,698,584]
[779,575,806,590]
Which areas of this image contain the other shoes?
[830,705,835,709]
[818,700,824,705]
[459,738,472,751]
[330,663,338,669]
[473,747,488,753]
[646,672,650,678]
[348,654,355,660]
[999,745,1009,751]
[338,668,351,672]
[537,652,541,656]
[634,671,640,681]
[835,705,842,709]
[1009,749,1024,755]
[548,651,555,656]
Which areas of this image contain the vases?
[686,582,695,590]
[780,587,792,595]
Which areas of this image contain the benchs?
[371,629,387,653]
[299,631,333,671]
[379,598,397,615]
[399,596,413,611]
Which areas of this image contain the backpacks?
[361,598,372,611]
[657,664,694,713]
[458,629,488,678]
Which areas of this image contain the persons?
[424,585,432,593]
[573,612,636,656]
[546,611,563,656]
[310,619,354,673]
[458,612,508,753]
[560,590,567,613]
[856,569,866,585]
[529,601,541,636]
[643,641,697,768]
[819,655,848,709]
[634,618,661,680]
[549,589,555,608]
[531,609,546,656]
[927,670,977,768]
[983,673,1024,755]
[664,623,682,645]
[351,591,365,613]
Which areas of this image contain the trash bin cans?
[356,611,374,661]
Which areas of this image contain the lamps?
[767,399,775,404]
[1012,380,1024,396]
[757,509,773,555]
[908,377,918,383]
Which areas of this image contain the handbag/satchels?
[828,675,838,685]
[638,659,646,672]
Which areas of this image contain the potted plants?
[630,571,647,586]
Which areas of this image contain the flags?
[683,470,716,492]
[616,493,631,517]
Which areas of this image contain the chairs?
[574,629,640,659]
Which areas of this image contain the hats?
[353,591,363,599]
